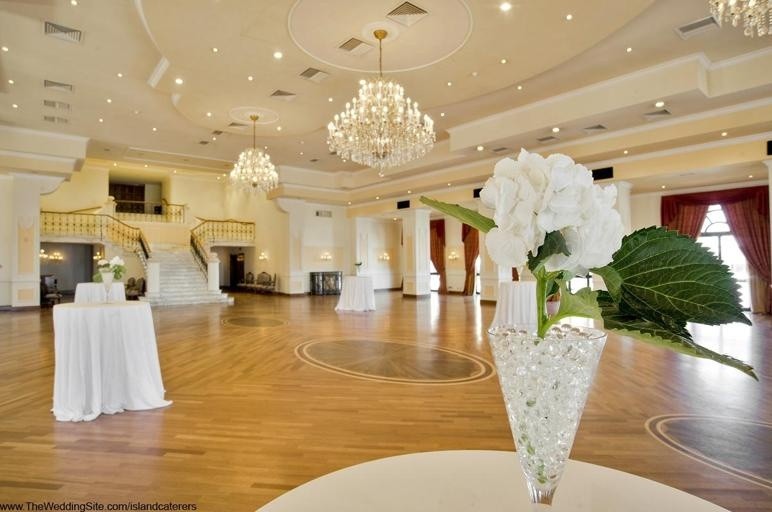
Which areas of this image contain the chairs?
[40,274,63,310]
[124,277,145,301]
[237,271,276,294]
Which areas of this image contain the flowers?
[90,251,129,286]
[418,145,760,380]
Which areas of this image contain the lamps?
[326,27,436,181]
[227,114,280,198]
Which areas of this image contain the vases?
[489,327,610,512]
[99,274,117,302]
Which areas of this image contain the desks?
[341,275,374,311]
[489,280,541,331]
[53,280,154,416]
[255,445,732,512]
